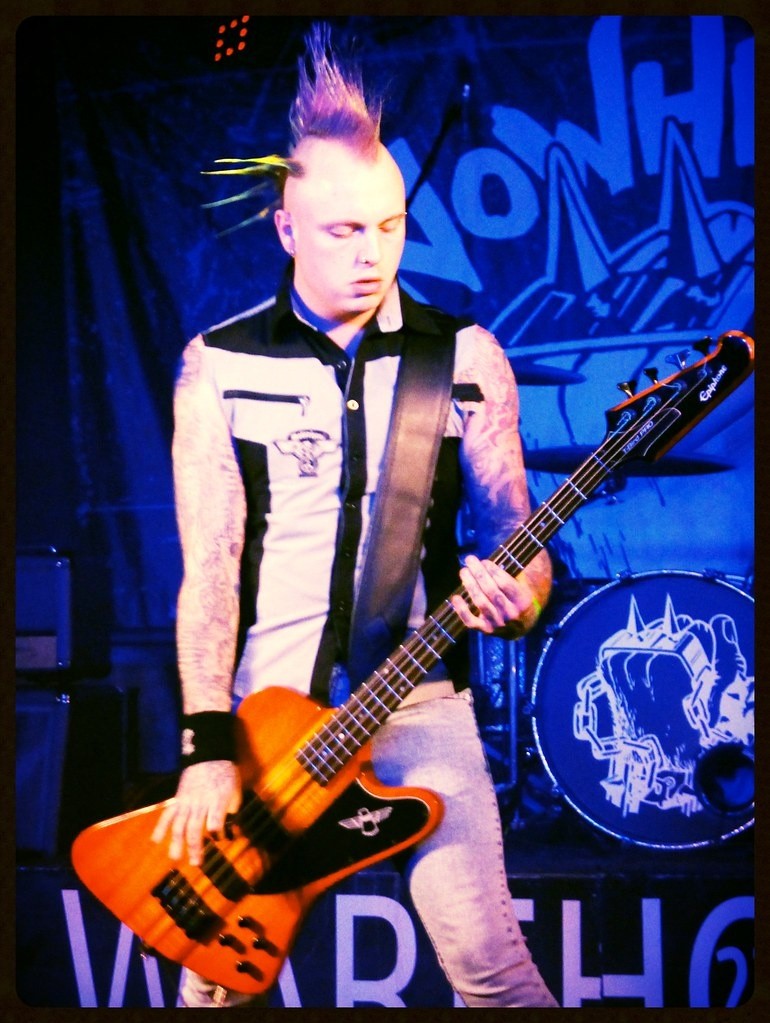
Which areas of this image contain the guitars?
[61,321,756,1004]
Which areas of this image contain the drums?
[525,566,755,853]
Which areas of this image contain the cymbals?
[521,437,738,479]
[503,356,589,389]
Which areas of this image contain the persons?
[148,37,563,1020]
